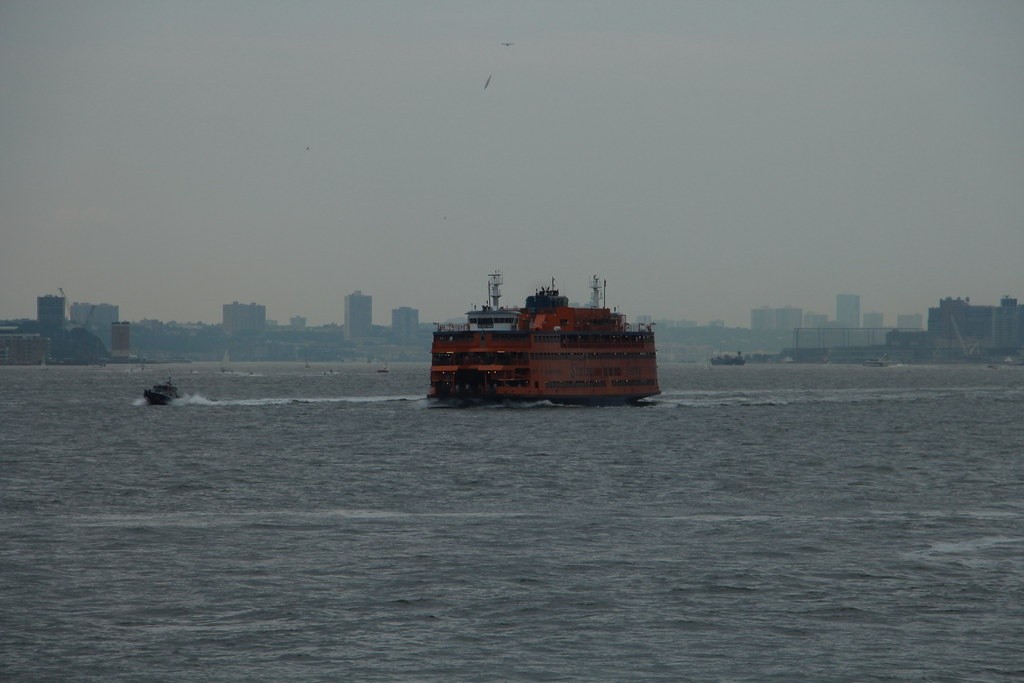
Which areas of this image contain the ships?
[424,269,663,409]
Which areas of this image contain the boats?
[710,354,746,365]
[143,376,178,405]
